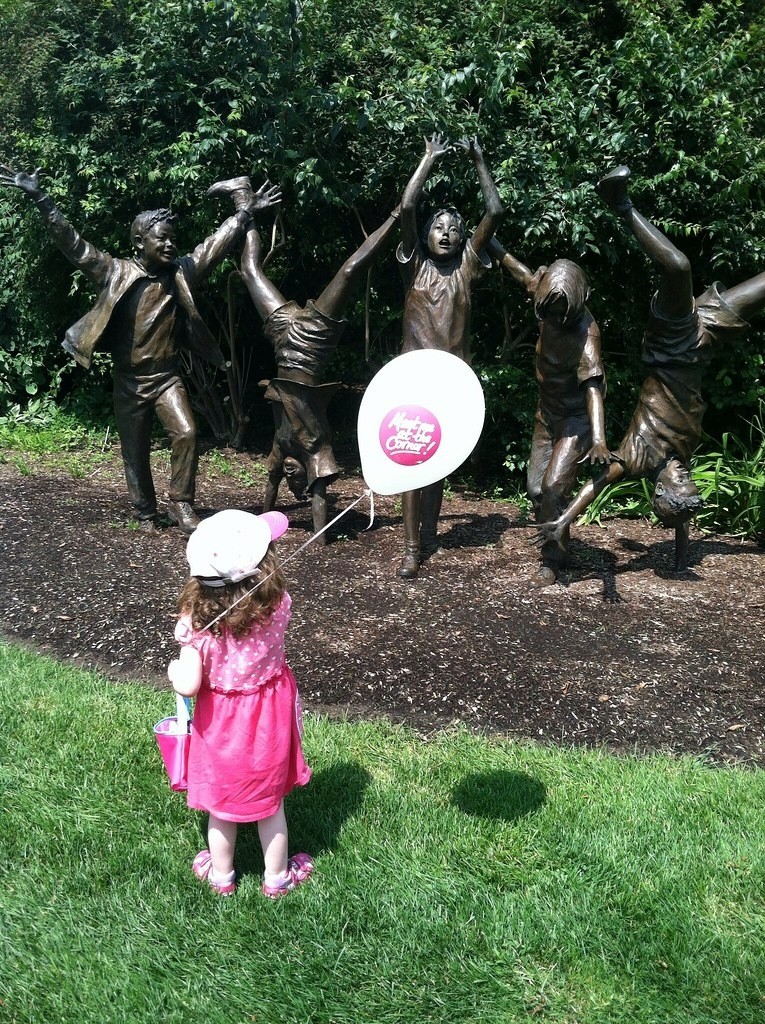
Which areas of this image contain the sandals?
[262,851,315,899]
[193,849,237,897]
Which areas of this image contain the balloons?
[359,343,488,497]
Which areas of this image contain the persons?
[521,168,765,570]
[468,192,628,583]
[210,175,426,547]
[0,154,289,538]
[168,506,323,903]
[391,123,509,581]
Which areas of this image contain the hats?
[185,508,289,587]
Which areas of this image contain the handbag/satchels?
[153,693,193,793]
